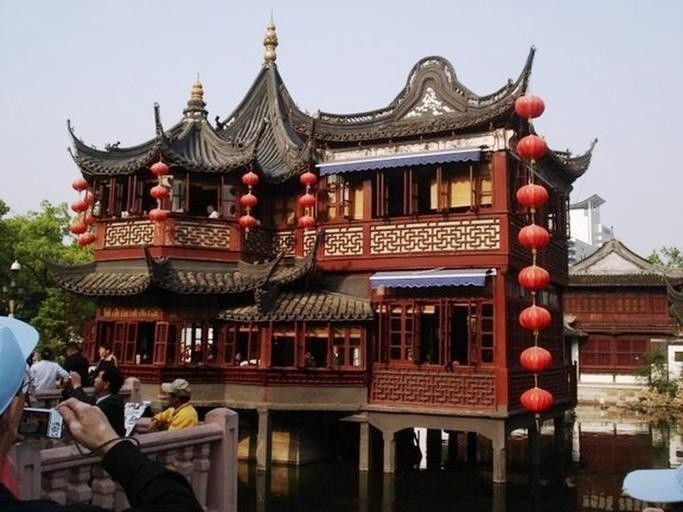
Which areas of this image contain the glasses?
[16,370,33,396]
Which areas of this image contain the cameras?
[18,407,65,439]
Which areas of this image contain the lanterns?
[298,164,316,231]
[71,174,96,246]
[148,153,170,221]
[517,77,555,430]
[240,162,260,241]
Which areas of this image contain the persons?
[30,347,70,391]
[135,350,151,364]
[180,343,213,364]
[147,378,199,431]
[88,342,118,388]
[27,351,38,366]
[333,345,341,365]
[206,205,218,218]
[62,368,127,437]
[306,351,316,366]
[0,316,205,511]
[63,342,89,387]
[176,198,185,211]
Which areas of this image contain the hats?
[161,379,191,397]
[0,315,40,415]
